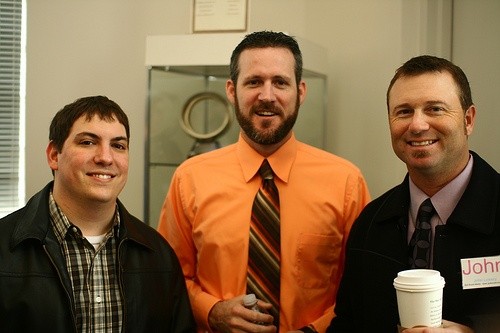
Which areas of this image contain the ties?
[245,159,281,333]
[406,197,436,269]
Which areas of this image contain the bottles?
[244,294,271,325]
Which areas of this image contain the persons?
[158,29,371,333]
[326,55,500,333]
[0,95,198,333]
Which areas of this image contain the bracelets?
[297,326,316,333]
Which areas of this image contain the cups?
[394,269,445,328]
